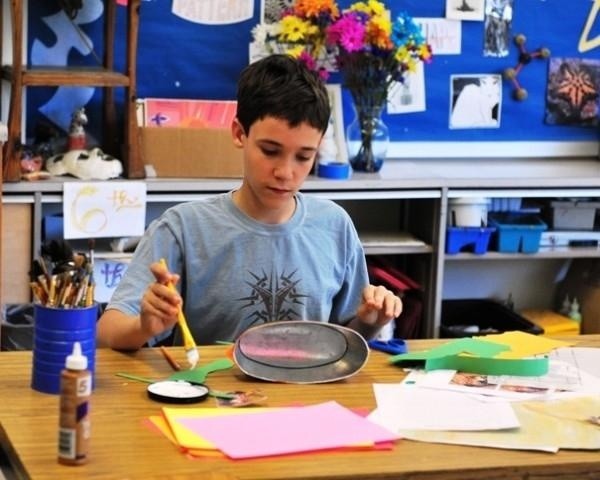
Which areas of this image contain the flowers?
[250,1,433,103]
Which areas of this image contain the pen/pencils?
[29,247,95,307]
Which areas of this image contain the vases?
[346,103,389,172]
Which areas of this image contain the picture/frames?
[543,56,599,130]
[448,74,503,129]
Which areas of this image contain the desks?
[1,334,600,480]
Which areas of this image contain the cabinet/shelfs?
[36,181,447,337]
[0,0,139,174]
[435,182,600,337]
[2,183,36,338]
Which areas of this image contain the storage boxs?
[488,212,548,253]
[446,227,495,255]
[137,126,246,179]
[550,200,598,229]
[518,308,579,334]
[485,198,523,211]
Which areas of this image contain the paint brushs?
[159,257,200,371]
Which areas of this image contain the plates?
[147,380,210,405]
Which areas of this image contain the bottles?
[561,294,582,326]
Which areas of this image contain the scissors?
[368,337,408,356]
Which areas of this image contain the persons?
[82,54,407,354]
